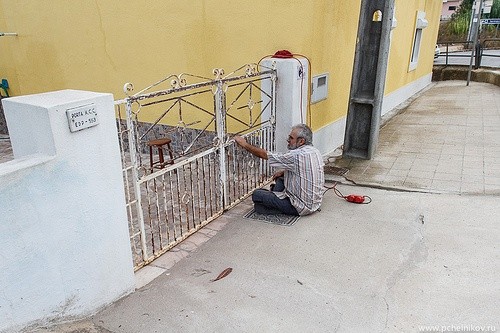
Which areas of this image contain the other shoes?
[254,204,282,214]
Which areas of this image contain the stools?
[147,137,177,174]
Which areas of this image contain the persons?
[232,124,326,216]
